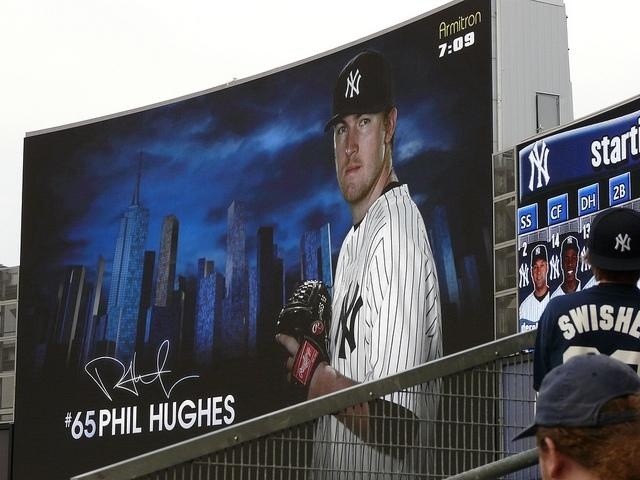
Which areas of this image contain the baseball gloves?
[275,278,332,398]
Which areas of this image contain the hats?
[586,207,640,272]
[510,352,640,442]
[530,236,580,262]
[324,50,398,132]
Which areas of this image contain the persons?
[518,244,551,335]
[508,351,639,478]
[530,206,640,395]
[548,234,582,301]
[271,48,447,480]
